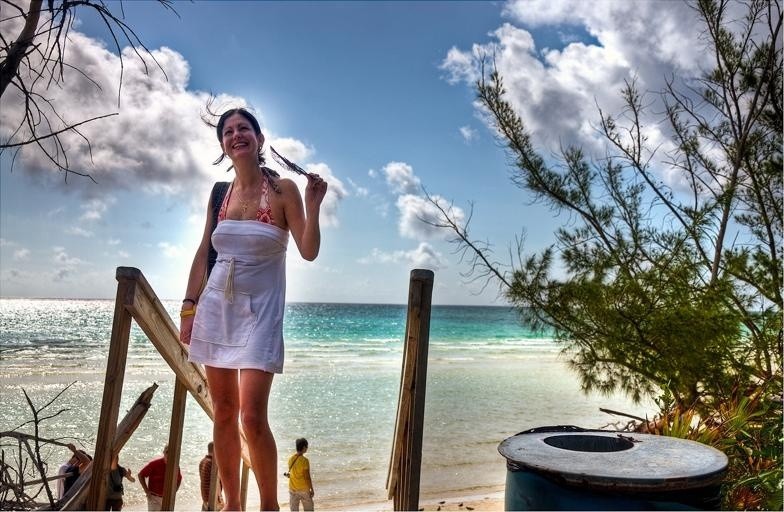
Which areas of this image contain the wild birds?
[418,501,474,512]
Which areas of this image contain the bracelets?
[183,298,195,304]
[180,305,196,318]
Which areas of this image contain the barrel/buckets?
[496,426,730,512]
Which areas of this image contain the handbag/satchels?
[113,483,123,492]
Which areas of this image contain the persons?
[64,454,92,511]
[197,440,226,511]
[107,455,135,511]
[287,437,315,511]
[137,446,182,511]
[57,450,86,500]
[179,104,327,512]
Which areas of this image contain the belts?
[151,493,162,498]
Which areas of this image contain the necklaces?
[234,172,263,213]
